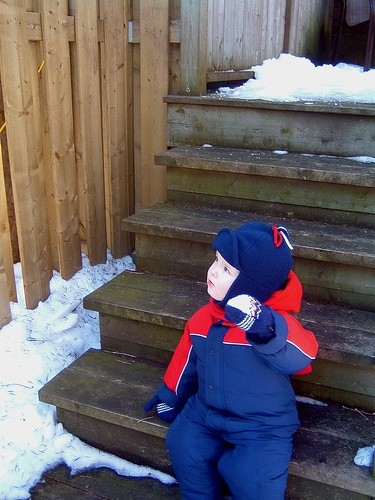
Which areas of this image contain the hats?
[214,222,294,309]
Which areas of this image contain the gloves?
[145,395,181,423]
[224,294,274,342]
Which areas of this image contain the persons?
[142,223,319,499]
